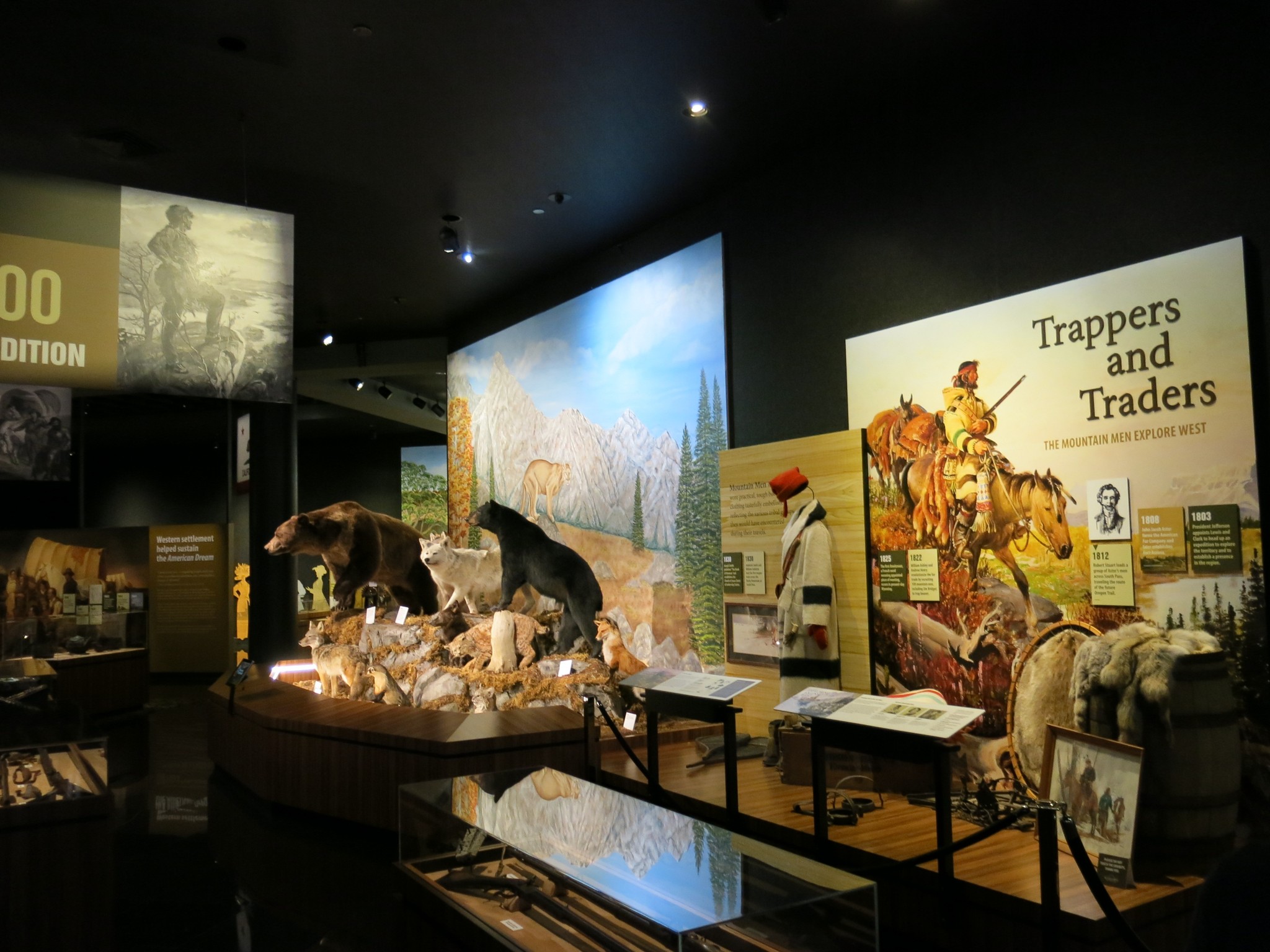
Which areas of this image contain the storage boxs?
[399,765,880,952]
[780,731,936,797]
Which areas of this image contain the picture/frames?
[1034,723,1144,868]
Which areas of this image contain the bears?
[264,501,437,616]
[465,499,603,658]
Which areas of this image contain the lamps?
[412,395,426,410]
[430,399,445,418]
[348,378,364,391]
[378,381,392,399]
[439,214,460,254]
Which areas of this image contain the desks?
[0,647,150,726]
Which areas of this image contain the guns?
[435,870,636,952]
[38,748,93,801]
